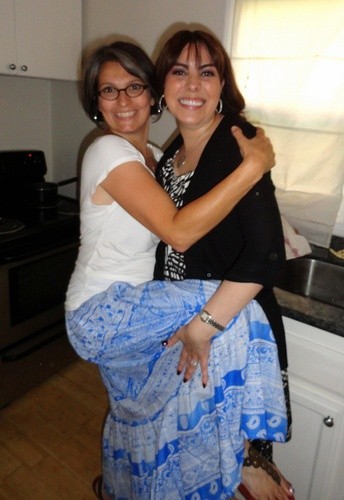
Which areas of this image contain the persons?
[63,41,295,500]
[150,31,292,500]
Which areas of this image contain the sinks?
[262,252,344,312]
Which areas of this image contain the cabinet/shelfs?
[0,2,84,81]
[273,314,344,500]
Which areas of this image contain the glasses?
[97,83,149,101]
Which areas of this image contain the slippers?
[237,446,295,500]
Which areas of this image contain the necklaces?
[175,120,215,170]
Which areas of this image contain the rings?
[189,360,198,367]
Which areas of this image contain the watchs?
[198,308,226,332]
